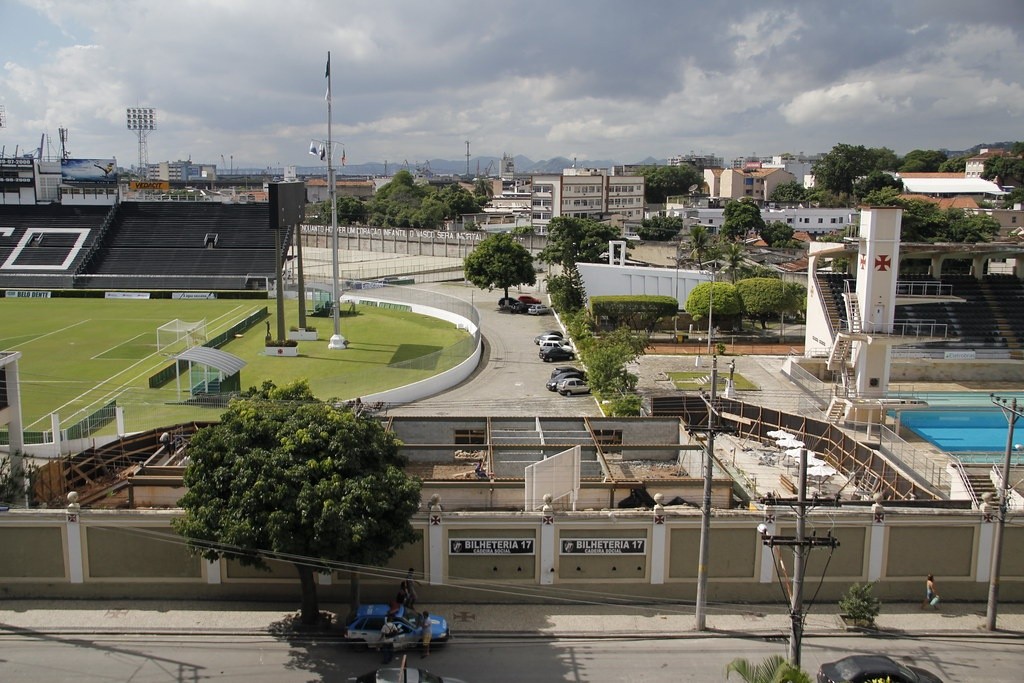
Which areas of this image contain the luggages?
[398,594,410,606]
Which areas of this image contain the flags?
[309,142,346,166]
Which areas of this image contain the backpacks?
[383,624,399,640]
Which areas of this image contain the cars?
[541,347,575,362]
[528,304,550,316]
[534,329,563,345]
[518,295,542,304]
[546,371,588,392]
[557,378,593,396]
[354,654,464,683]
[816,654,944,683]
[540,340,573,353]
[342,604,450,651]
[498,297,519,310]
[550,367,586,382]
[510,302,531,314]
[539,336,564,345]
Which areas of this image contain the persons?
[726,359,735,379]
[379,618,398,665]
[419,611,431,658]
[920,574,938,610]
[94,163,114,177]
[397,569,421,611]
[160,431,171,446]
[265,319,271,336]
[841,258,847,273]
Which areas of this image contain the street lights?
[127,107,157,202]
